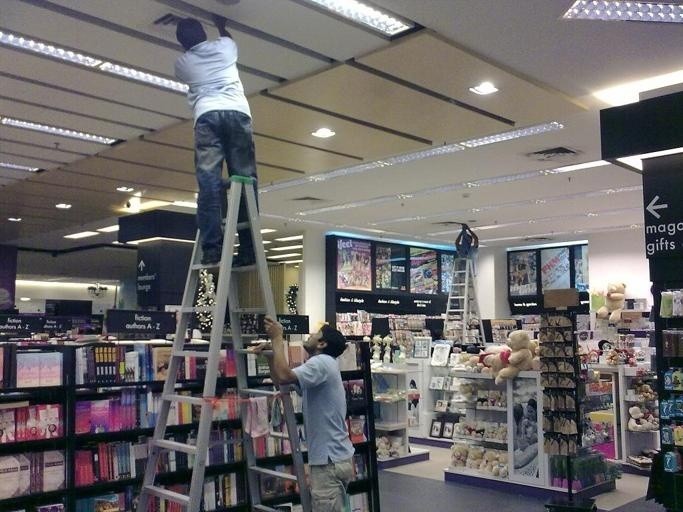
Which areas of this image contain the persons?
[455,223,478,314]
[514,399,540,475]
[263,316,356,506]
[171,16,259,264]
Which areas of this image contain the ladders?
[137,175,313,512]
[441,256,486,346]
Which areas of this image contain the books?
[259,464,314,499]
[335,380,365,412]
[0,404,64,441]
[74,423,248,486]
[250,421,308,459]
[347,415,367,445]
[72,389,304,434]
[343,455,367,481]
[0,453,66,498]
[74,344,285,385]
[288,344,364,377]
[344,493,368,507]
[76,465,247,504]
[1,342,65,390]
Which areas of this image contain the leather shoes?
[231,246,256,268]
[200,252,221,265]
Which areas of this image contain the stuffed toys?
[629,404,662,431]
[629,377,657,403]
[595,281,627,323]
[492,329,533,385]
[454,381,507,409]
[468,345,510,374]
[457,418,508,440]
[451,445,509,479]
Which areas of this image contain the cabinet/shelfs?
[484,311,617,349]
[326,311,485,354]
[0,339,379,512]
[659,284,683,512]
[405,353,450,448]
[371,365,409,469]
[538,307,621,512]
[442,366,543,498]
[581,308,658,478]
